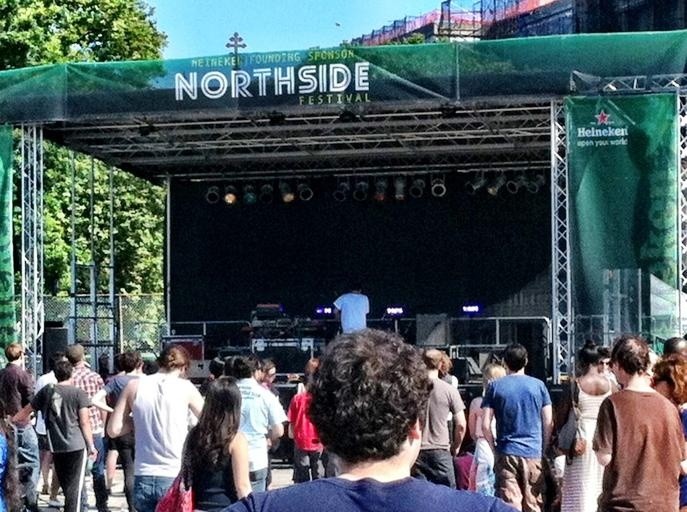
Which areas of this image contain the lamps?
[193,166,324,206]
[331,168,452,201]
[456,165,550,195]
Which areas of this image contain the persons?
[411,334,687,511]
[333,284,370,334]
[0,342,338,511]
[222,330,520,511]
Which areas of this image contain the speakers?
[164,334,213,386]
[38,326,67,374]
[516,322,546,388]
[415,311,449,350]
[251,338,314,388]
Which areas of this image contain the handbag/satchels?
[155,469,194,512]
[553,408,586,458]
